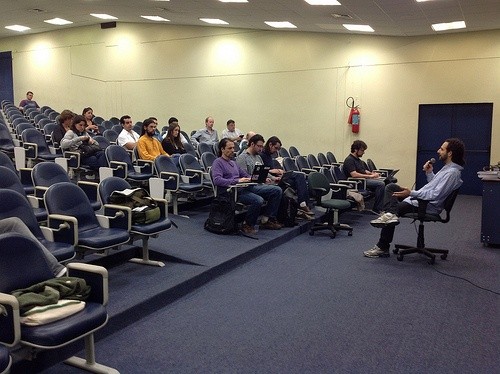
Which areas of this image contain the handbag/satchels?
[77,143,102,157]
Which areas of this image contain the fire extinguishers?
[346,96,360,133]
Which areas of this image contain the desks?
[480,176,500,247]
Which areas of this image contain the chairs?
[0,97,458,374]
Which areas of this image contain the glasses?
[256,144,263,148]
[274,146,281,151]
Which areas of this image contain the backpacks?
[106,186,160,226]
[204,196,236,235]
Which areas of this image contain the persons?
[239,132,256,149]
[118,115,140,149]
[19,91,40,108]
[149,117,163,142]
[137,119,175,161]
[162,117,188,144]
[222,120,244,146]
[343,140,386,215]
[52,110,75,142]
[60,115,107,171]
[82,107,101,135]
[363,138,465,257]
[235,134,285,227]
[162,123,184,155]
[190,117,220,146]
[211,138,282,238]
[260,136,316,220]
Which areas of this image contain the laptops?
[377,170,399,181]
[241,165,271,183]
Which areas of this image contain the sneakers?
[363,245,390,258]
[369,213,400,228]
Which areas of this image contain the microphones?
[423,158,436,171]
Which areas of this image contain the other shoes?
[296,204,314,215]
[297,212,315,221]
[259,220,281,230]
[237,222,259,240]
